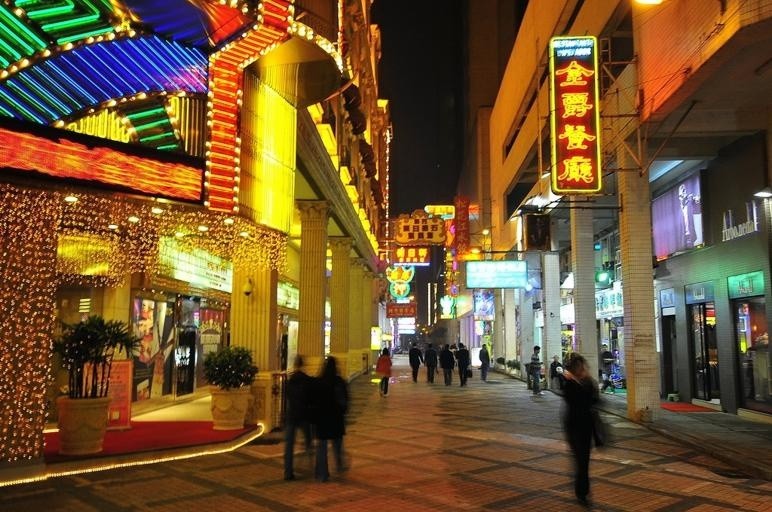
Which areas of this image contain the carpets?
[662,403,720,413]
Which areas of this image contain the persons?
[374,347,393,399]
[309,357,350,481]
[557,352,602,510]
[407,339,491,389]
[530,346,544,398]
[550,356,562,390]
[601,343,618,395]
[280,355,314,478]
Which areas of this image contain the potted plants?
[202,344,260,432]
[54,316,144,459]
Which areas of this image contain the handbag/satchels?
[590,409,608,447]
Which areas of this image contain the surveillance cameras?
[242,283,252,296]
